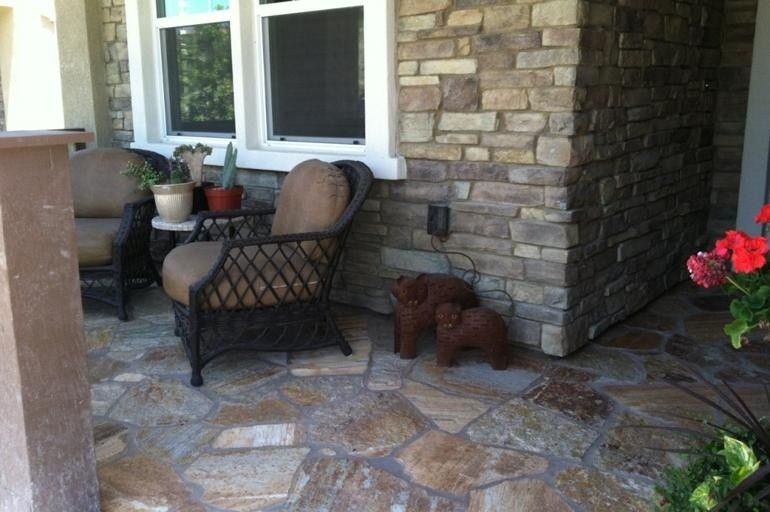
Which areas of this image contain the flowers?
[687,202,769,350]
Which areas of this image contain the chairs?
[162,159,373,386]
[67,147,170,322]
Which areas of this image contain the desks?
[150,206,253,280]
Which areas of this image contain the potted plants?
[205,142,243,213]
[173,142,214,214]
[119,160,197,224]
